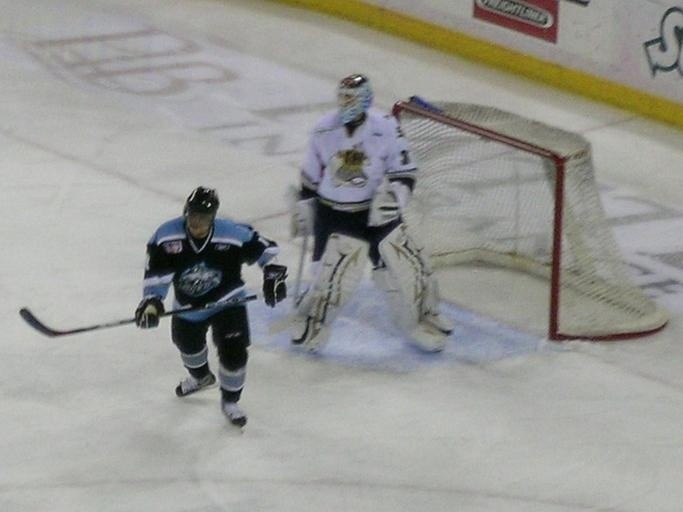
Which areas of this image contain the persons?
[287,70,455,359]
[132,185,288,425]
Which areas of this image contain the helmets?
[335,73,373,124]
[183,186,219,228]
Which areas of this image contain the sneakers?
[175,372,216,398]
[222,399,247,427]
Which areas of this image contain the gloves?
[134,297,165,330]
[291,195,317,239]
[262,263,288,309]
[367,170,416,227]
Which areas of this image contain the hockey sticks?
[21,294,258,337]
